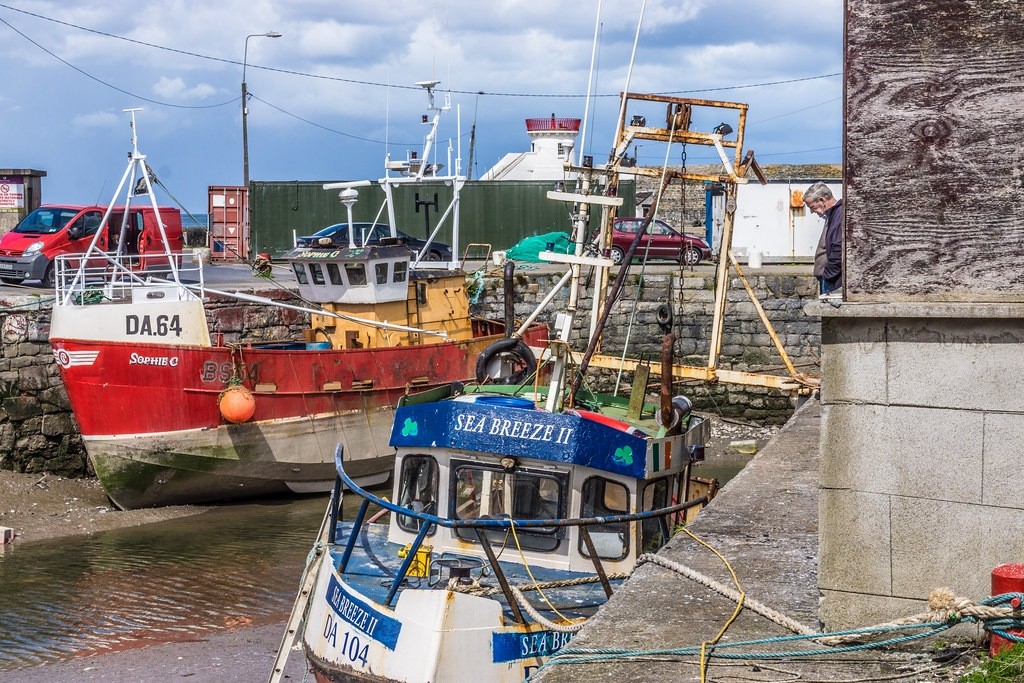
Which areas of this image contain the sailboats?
[267,0,714,683]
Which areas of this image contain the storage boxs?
[405,542,434,578]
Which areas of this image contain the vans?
[0,203,183,288]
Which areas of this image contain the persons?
[802,182,844,294]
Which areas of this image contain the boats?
[385,81,639,182]
[46,107,550,511]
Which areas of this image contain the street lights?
[468,92,483,178]
[243,33,281,186]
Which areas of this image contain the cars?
[296,222,452,278]
[590,217,711,272]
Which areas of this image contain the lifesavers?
[472,336,537,386]
[654,302,672,326]
